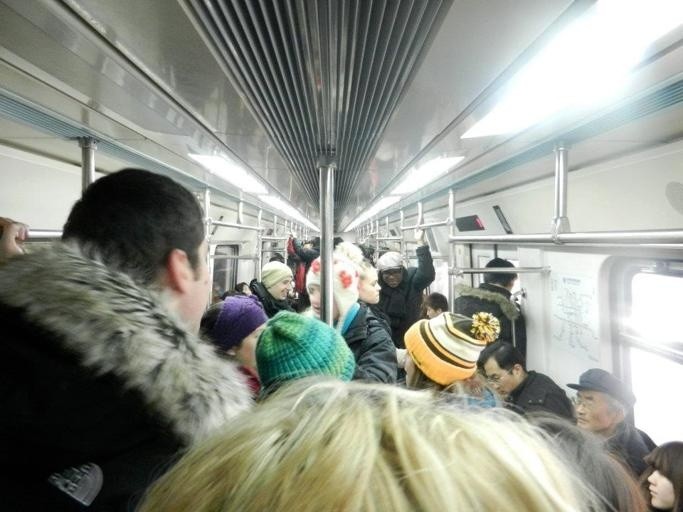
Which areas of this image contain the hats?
[305,241,362,334]
[261,261,293,290]
[566,369,634,410]
[255,311,355,395]
[403,311,500,384]
[377,252,402,271]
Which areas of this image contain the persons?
[522,411,683,512]
[197,220,651,479]
[0,168,252,512]
[133,367,618,512]
